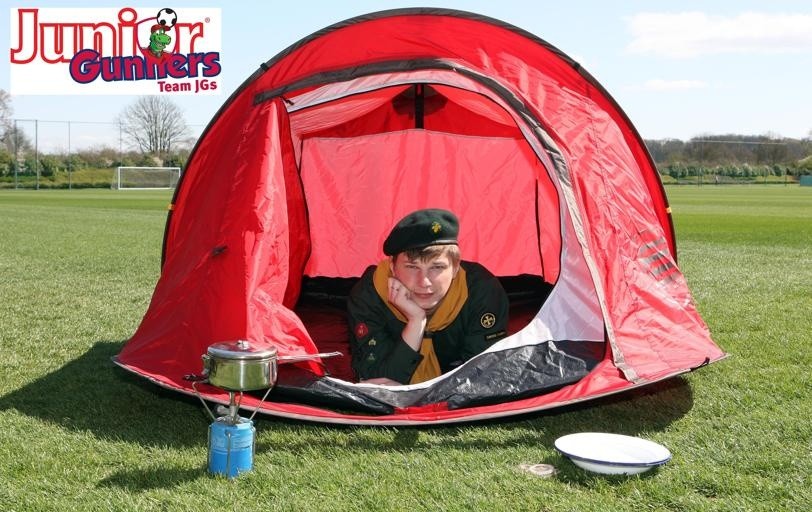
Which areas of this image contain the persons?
[348,208,509,386]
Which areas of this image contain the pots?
[184,342,343,392]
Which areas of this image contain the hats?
[382,208,459,256]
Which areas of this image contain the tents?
[110,6,731,426]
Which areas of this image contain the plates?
[557,433,670,474]
